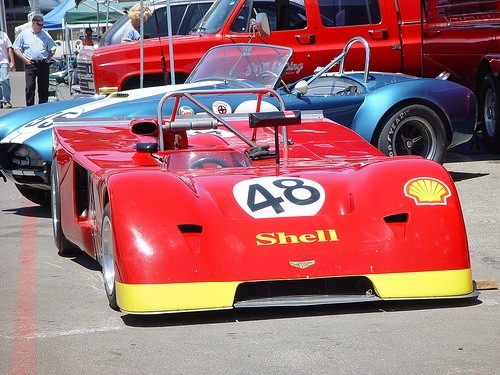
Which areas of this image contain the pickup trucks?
[76,0,500,154]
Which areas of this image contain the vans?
[71,0,338,95]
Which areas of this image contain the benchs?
[291,77,364,96]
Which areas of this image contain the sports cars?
[0,36,478,207]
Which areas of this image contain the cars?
[50,88,481,315]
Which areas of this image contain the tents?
[41,0,82,65]
[61,0,145,99]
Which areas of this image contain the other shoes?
[4,102,12,108]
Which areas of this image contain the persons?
[83,28,94,46]
[98,27,106,38]
[13,15,56,107]
[0,31,16,108]
[121,3,152,43]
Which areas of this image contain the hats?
[32,15,46,25]
[85,28,94,32]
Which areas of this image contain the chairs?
[167,133,233,170]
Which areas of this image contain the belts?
[31,59,46,63]
